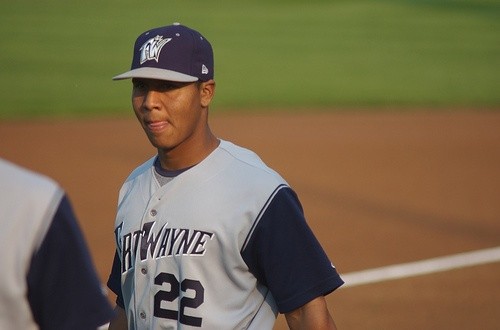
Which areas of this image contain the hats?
[111,22,215,85]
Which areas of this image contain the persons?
[1,159,116,330]
[108,23,345,330]
